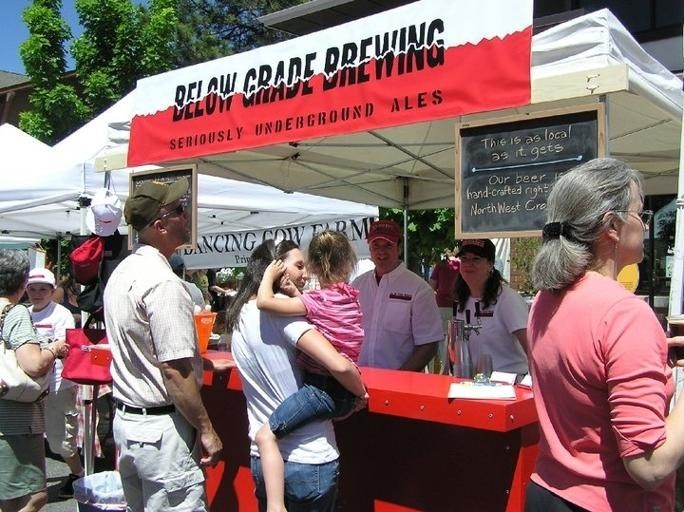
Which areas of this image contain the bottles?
[201,305,211,312]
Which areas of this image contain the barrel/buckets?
[192,313,217,353]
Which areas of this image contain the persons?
[256,231,369,512]
[102,177,226,512]
[450,239,533,382]
[226,237,370,512]
[350,218,446,375]
[0,221,237,512]
[524,156,683,512]
[429,250,460,372]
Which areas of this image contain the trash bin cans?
[73,471,129,512]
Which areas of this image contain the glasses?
[615,209,653,226]
[149,202,185,227]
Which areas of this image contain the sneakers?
[58,473,81,498]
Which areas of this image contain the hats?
[25,266,58,289]
[91,229,123,260]
[124,176,189,232]
[455,239,495,262]
[367,220,400,248]
[68,237,104,284]
[75,278,104,322]
[86,188,123,237]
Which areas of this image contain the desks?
[191,347,551,512]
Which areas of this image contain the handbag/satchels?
[60,328,112,385]
[0,341,49,405]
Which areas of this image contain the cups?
[666,315,684,361]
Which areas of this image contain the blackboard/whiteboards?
[454,102,606,240]
[127,163,197,251]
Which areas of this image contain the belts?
[118,402,175,415]
